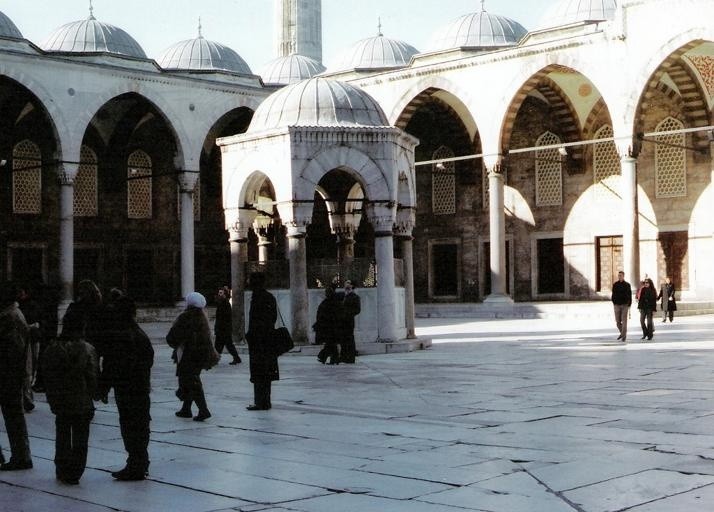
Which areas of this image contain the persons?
[611,270,632,342]
[316,283,354,365]
[637,278,657,341]
[338,279,360,364]
[0,276,154,486]
[656,276,677,323]
[634,280,655,333]
[215,287,241,365]
[245,271,276,411]
[165,292,219,422]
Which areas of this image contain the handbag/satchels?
[203,341,221,370]
[271,325,294,356]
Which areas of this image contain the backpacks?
[37,339,72,387]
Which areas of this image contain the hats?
[185,291,206,308]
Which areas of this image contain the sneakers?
[641,335,648,339]
[1,458,33,470]
[621,336,626,341]
[174,409,191,418]
[110,466,150,480]
[227,358,241,366]
[193,410,211,421]
[55,469,80,485]
[647,335,653,340]
[617,335,622,339]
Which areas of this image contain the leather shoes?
[245,403,271,410]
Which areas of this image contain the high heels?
[326,360,340,366]
[316,357,325,364]
